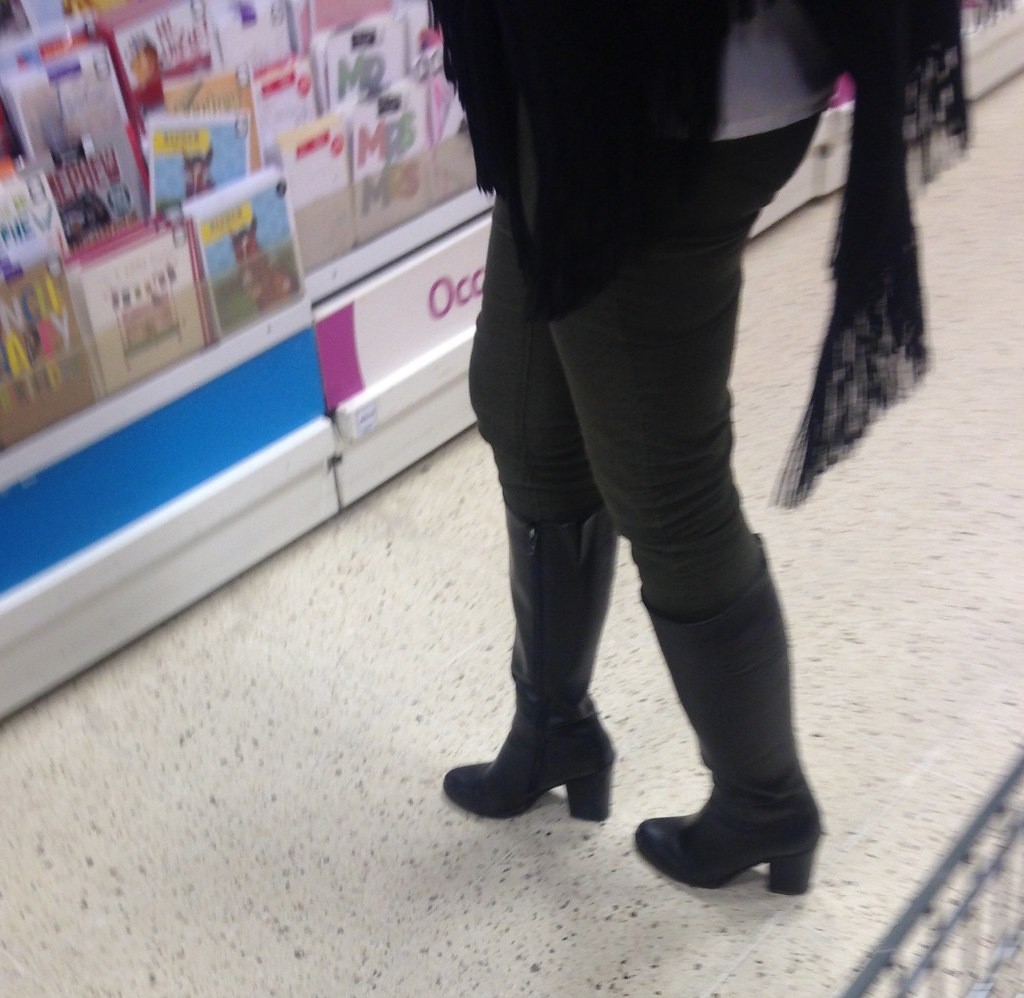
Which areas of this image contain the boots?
[635,533,820,895]
[442,502,621,821]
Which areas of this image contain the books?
[0,0,476,450]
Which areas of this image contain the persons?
[423,1,971,893]
